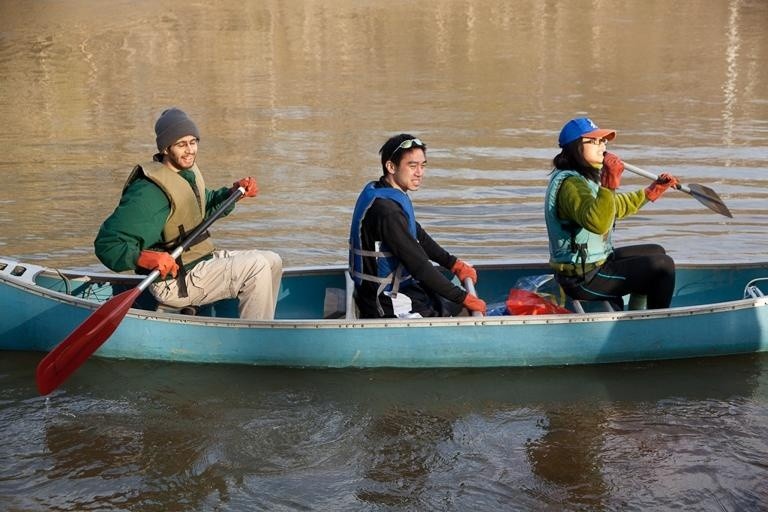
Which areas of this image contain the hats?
[154,108,200,155]
[558,117,615,147]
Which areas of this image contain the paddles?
[603,151,732,219]
[36,178,248,395]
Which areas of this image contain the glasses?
[389,138,423,160]
[582,138,608,146]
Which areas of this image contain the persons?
[350,135,486,317]
[546,117,675,311]
[94,109,284,321]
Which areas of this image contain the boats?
[0,257,768,370]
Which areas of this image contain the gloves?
[230,176,258,202]
[601,152,624,189]
[463,291,486,316]
[136,249,179,280]
[644,172,678,203]
[450,258,477,285]
